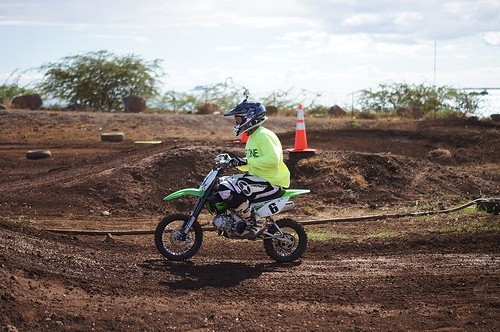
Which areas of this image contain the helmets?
[223,99,268,136]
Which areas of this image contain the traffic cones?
[287,104,317,153]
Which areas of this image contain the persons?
[214,101,290,241]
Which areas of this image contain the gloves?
[228,158,247,166]
[213,152,231,164]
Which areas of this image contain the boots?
[230,194,267,239]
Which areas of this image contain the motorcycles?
[154,151,322,261]
